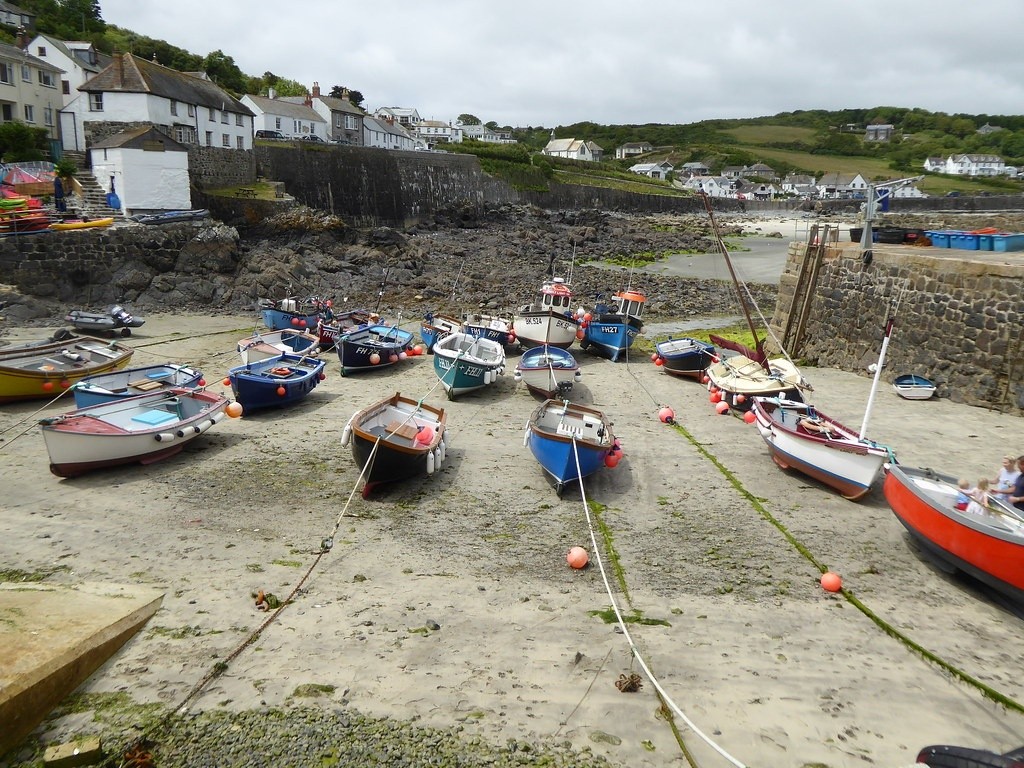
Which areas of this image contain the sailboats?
[698,178,815,414]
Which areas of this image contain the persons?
[991,456,1024,512]
[990,454,1022,506]
[957,475,990,515]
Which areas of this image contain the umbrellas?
[53,177,67,219]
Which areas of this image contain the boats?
[653,334,715,384]
[526,397,615,496]
[227,351,326,414]
[316,266,391,347]
[747,278,914,503]
[128,207,212,225]
[64,304,146,336]
[46,216,114,231]
[463,306,514,348]
[330,323,414,377]
[0,324,136,403]
[235,326,321,366]
[516,344,580,399]
[881,462,1023,621]
[510,238,582,351]
[73,360,205,410]
[892,373,936,400]
[0,181,63,236]
[419,312,463,356]
[350,390,447,501]
[255,281,335,333]
[432,328,506,400]
[37,381,230,478]
[579,258,647,363]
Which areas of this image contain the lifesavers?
[863,250,872,263]
[552,284,568,292]
[271,367,290,374]
[628,291,643,295]
[800,419,835,433]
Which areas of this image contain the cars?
[330,139,352,145]
[299,134,325,142]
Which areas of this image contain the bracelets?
[1017,497,1019,501]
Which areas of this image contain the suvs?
[255,129,285,139]
